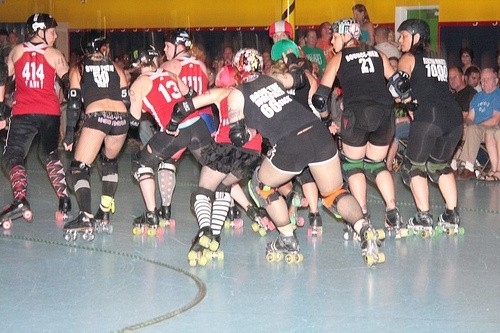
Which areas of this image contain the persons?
[0,3,500,251]
[227,48,376,259]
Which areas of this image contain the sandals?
[479,169,500,181]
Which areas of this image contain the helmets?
[27,12,58,32]
[397,18,430,42]
[215,64,241,86]
[235,47,263,74]
[269,20,294,40]
[166,28,192,50]
[80,28,110,56]
[137,47,161,65]
[331,17,362,41]
[271,39,299,61]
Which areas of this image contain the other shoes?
[452,168,476,180]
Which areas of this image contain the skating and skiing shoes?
[435,206,465,235]
[132,209,165,237]
[56,197,74,222]
[308,212,323,238]
[247,206,276,236]
[187,226,221,266]
[287,189,308,228]
[92,208,112,234]
[343,216,371,241]
[360,221,386,264]
[63,211,96,241]
[405,210,438,239]
[224,205,243,229]
[156,204,176,228]
[0,195,33,229]
[266,233,304,264]
[384,207,408,239]
